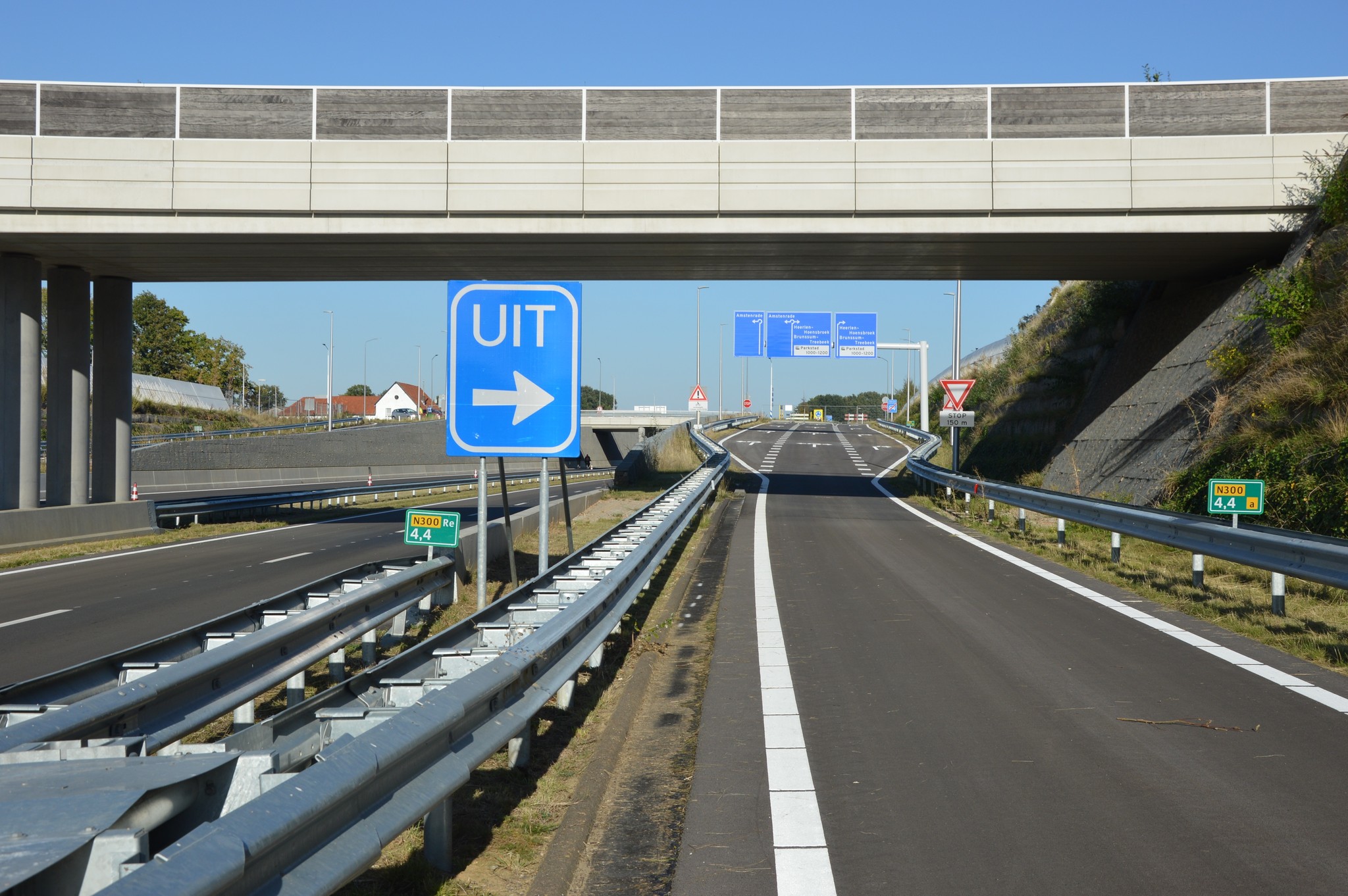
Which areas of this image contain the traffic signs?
[733,310,764,358]
[835,312,879,358]
[442,281,583,458]
[766,311,832,358]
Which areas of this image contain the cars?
[422,409,445,418]
[391,408,418,421]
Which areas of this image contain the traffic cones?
[130,482,139,501]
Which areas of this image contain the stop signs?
[744,400,752,408]
[881,402,887,412]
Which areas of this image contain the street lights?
[903,338,916,395]
[324,310,332,432]
[415,345,421,419]
[902,328,910,421]
[943,291,954,447]
[363,337,382,419]
[597,358,602,406]
[695,286,711,425]
[258,378,267,415]
[322,343,329,416]
[430,355,439,409]
[610,375,615,414]
[719,322,729,421]
